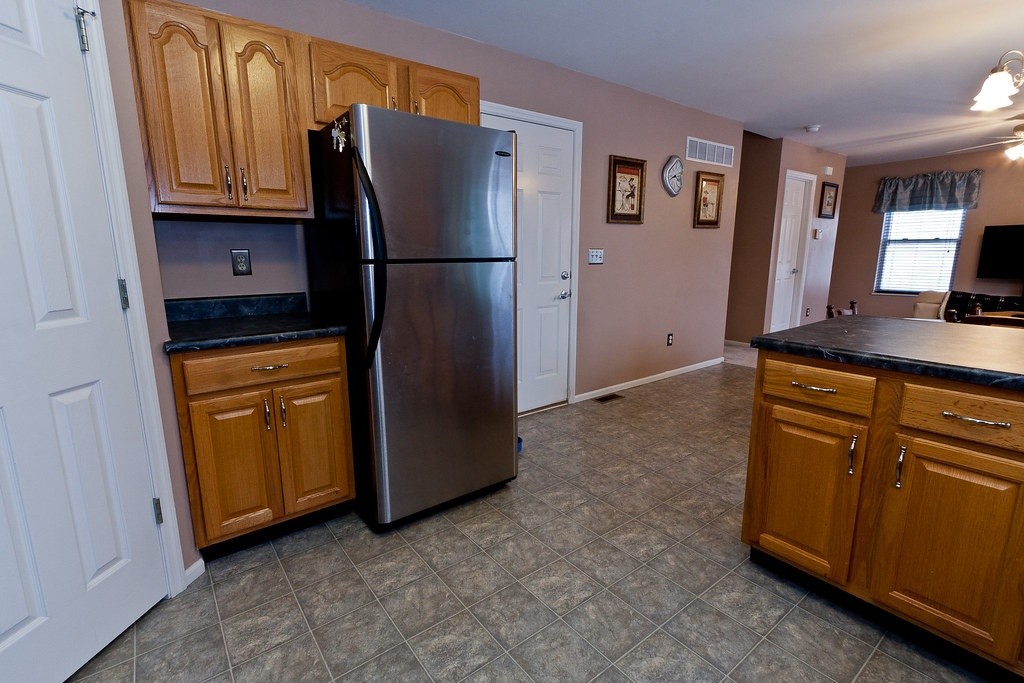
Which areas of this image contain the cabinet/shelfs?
[740,350,1024,678]
[169,336,355,549]
[309,35,480,131]
[121,0,314,219]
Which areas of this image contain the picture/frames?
[818,181,839,219]
[606,155,648,224]
[692,171,726,229]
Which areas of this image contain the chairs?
[825,300,858,318]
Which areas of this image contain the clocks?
[663,154,685,197]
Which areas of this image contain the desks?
[965,311,1024,328]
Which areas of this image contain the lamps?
[804,125,821,133]
[970,49,1024,160]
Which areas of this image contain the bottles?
[975,303,982,316]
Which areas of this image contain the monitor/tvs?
[976,224,1024,282]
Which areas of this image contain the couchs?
[914,291,1024,323]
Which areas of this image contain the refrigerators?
[310,104,520,536]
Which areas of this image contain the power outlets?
[589,247,603,265]
[231,249,253,276]
